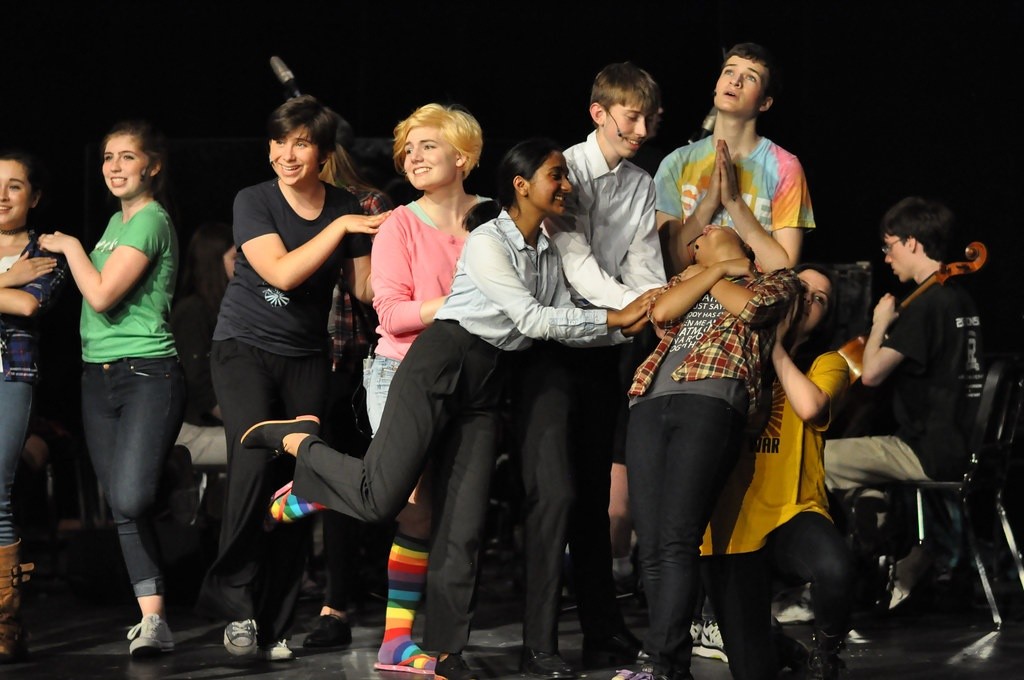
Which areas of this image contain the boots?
[806,630,849,680]
[0,537,34,663]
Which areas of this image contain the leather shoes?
[519,646,579,679]
[582,629,653,669]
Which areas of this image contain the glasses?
[687,234,703,264]
[882,237,904,255]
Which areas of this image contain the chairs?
[839,355,1024,631]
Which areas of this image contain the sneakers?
[127,614,175,657]
[241,415,321,453]
[223,619,257,655]
[888,544,934,611]
[302,613,353,648]
[434,653,479,680]
[259,638,295,660]
[698,620,728,663]
[690,619,704,654]
[775,605,815,626]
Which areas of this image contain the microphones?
[140,167,149,181]
[617,128,621,137]
[695,245,699,250]
[713,92,716,96]
[269,157,273,164]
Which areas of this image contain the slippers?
[374,654,435,674]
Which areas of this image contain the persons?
[772,191,984,625]
[242,44,851,680]
[0,148,67,662]
[37,118,178,654]
[172,97,391,662]
